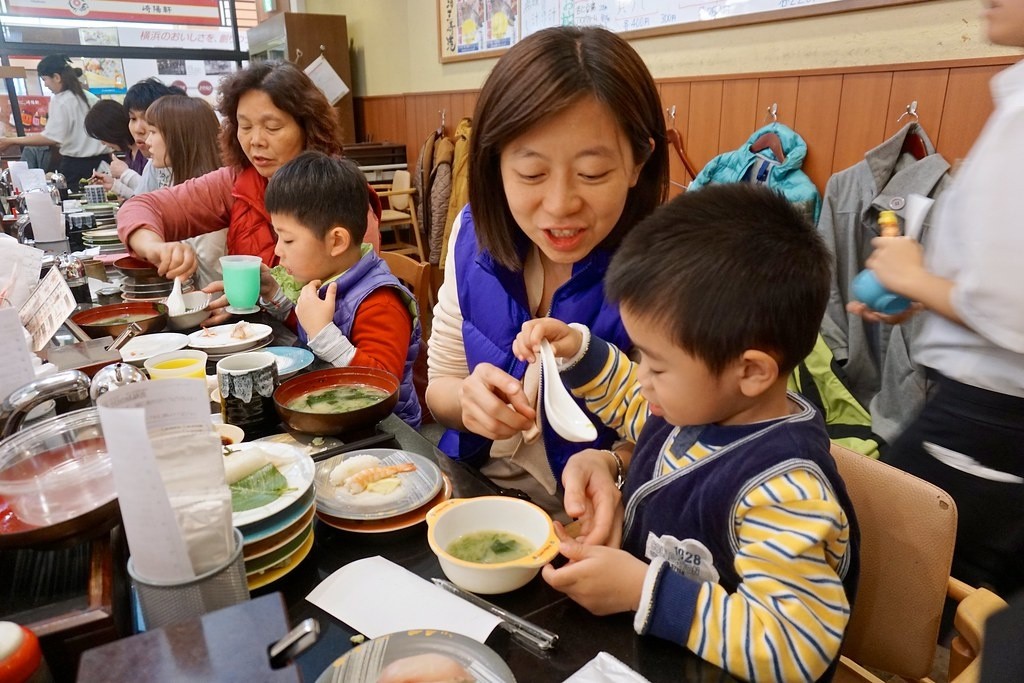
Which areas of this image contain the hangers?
[665,113,697,189]
[749,112,786,164]
[889,113,928,183]
[435,109,445,140]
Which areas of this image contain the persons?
[845,0,1024,683]
[511,182,861,683]
[425,26,673,550]
[0,53,114,182]
[260,151,422,432]
[83,80,189,195]
[21,143,61,173]
[145,96,229,298]
[118,62,380,344]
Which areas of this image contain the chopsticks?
[87,172,109,184]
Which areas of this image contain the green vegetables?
[304,386,390,414]
[489,538,518,552]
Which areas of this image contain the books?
[303,55,349,107]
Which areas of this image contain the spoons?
[167,279,187,314]
[540,337,597,441]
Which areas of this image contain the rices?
[329,455,380,486]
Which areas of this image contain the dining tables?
[0,257,746,683]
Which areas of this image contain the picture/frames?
[436,0,521,64]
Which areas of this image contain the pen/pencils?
[499,622,551,650]
[431,577,560,645]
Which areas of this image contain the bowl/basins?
[156,287,214,332]
[426,493,563,595]
[274,366,401,436]
[72,302,170,340]
[1,407,117,528]
[113,256,159,277]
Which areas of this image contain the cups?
[219,255,261,314]
[68,212,96,231]
[83,185,107,203]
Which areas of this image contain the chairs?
[370,171,424,289]
[379,249,431,403]
[829,443,1008,683]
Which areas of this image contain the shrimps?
[347,463,417,495]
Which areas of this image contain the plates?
[315,630,518,683]
[0,158,566,598]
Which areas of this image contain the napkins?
[305,555,503,646]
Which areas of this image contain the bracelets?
[602,449,626,490]
